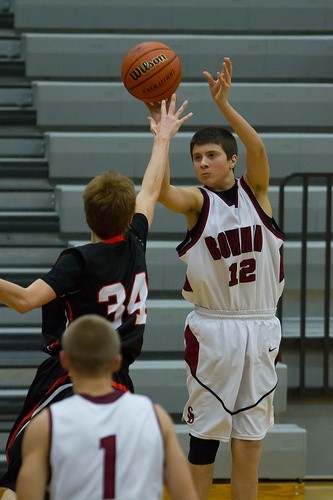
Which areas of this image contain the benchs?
[6,0,332,486]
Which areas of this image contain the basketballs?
[121,41,182,102]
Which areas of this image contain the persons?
[0,315,202,499]
[144,54,289,499]
[0,94,193,499]
